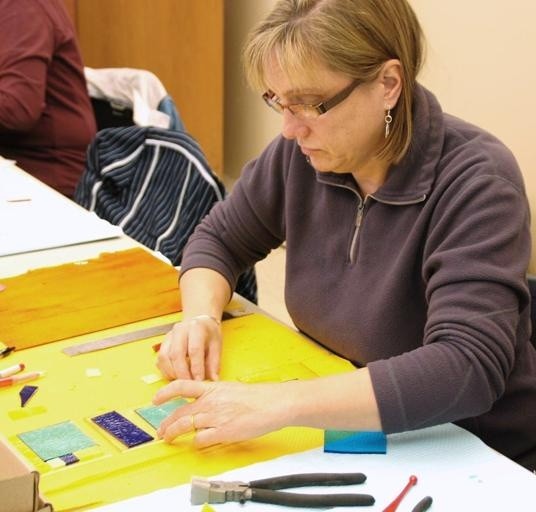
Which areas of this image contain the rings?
[189,413,196,431]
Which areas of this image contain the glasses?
[261,76,362,121]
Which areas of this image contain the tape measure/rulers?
[61,307,255,357]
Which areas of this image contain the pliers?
[190,473,375,507]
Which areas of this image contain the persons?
[151,0,536,473]
[0,1,97,204]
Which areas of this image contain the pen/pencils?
[411,495,433,512]
[0,347,46,388]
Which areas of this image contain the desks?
[0,154,536,512]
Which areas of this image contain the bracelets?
[181,314,223,329]
